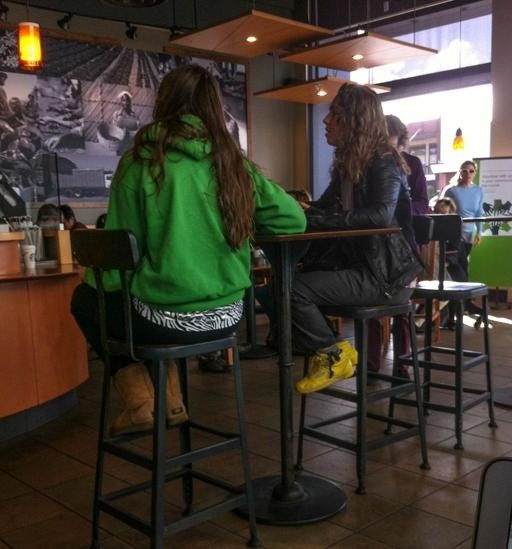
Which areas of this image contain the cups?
[253,248,266,265]
[24,269,37,277]
[20,244,37,270]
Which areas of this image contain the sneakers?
[336,340,358,365]
[296,350,355,394]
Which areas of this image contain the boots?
[166,360,189,425]
[108,364,154,436]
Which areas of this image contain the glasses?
[462,170,475,173]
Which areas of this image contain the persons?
[255,185,317,346]
[431,196,466,330]
[32,202,58,229]
[261,78,422,395]
[111,90,141,130]
[70,60,308,440]
[364,114,432,388]
[0,67,84,188]
[441,159,489,328]
[56,204,92,231]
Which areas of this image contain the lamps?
[16,0,43,70]
[122,22,178,42]
[450,3,465,150]
[55,11,73,32]
